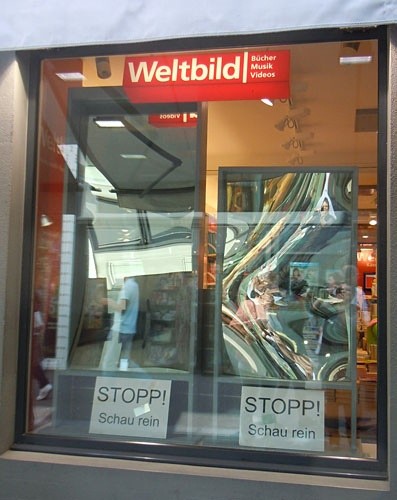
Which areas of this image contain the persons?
[287,266,309,300]
[91,269,142,371]
[313,263,378,389]
[31,269,54,402]
[228,269,311,384]
[299,196,338,239]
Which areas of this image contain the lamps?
[96,57,112,79]
[339,42,360,57]
[288,156,304,165]
[281,138,298,151]
[275,117,294,131]
[260,98,287,107]
